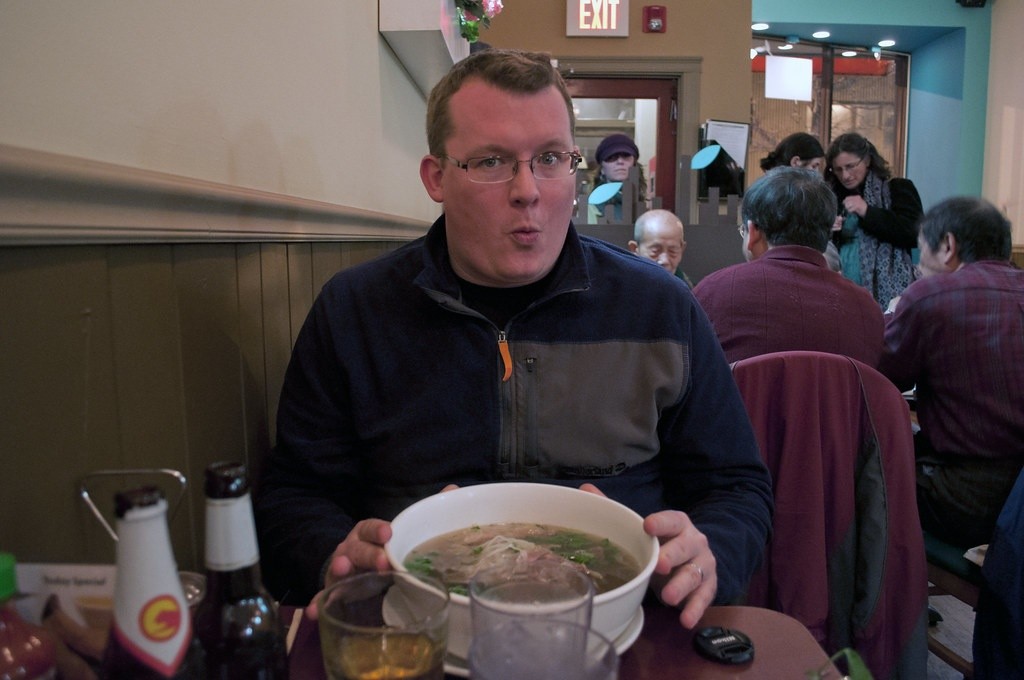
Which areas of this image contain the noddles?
[401,520,642,604]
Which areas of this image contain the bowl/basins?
[386,483,658,662]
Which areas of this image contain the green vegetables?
[401,526,630,597]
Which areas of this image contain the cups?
[470,562,619,680]
[318,572,449,680]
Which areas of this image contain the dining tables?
[285,604,845,680]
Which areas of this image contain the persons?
[693,165,885,371]
[582,133,654,219]
[760,132,824,174]
[882,195,1024,550]
[260,46,774,623]
[823,132,924,310]
[628,209,698,289]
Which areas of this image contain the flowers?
[457,0,507,44]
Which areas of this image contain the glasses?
[444,151,582,185]
[829,157,864,173]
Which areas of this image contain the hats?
[595,134,639,164]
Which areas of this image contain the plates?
[440,607,645,677]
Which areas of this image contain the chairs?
[728,350,926,680]
[925,529,981,680]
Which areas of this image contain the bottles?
[97,486,203,679]
[193,462,291,679]
[0,552,61,679]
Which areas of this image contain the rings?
[685,564,704,583]
